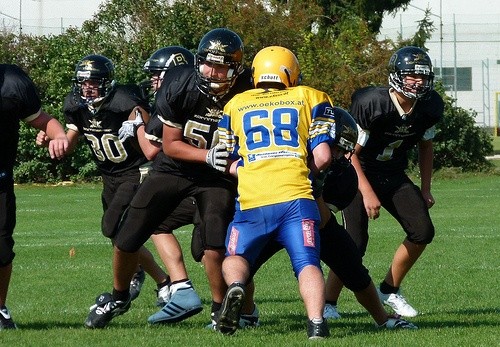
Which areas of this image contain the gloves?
[118,108,145,143]
[205,143,228,173]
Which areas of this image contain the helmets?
[332,106,359,154]
[252,45,299,89]
[140,45,194,98]
[72,54,115,104]
[387,48,433,100]
[196,29,244,99]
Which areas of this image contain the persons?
[322,47,446,316]
[34,28,418,339]
[0,63,70,328]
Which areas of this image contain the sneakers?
[210,306,219,331]
[306,317,330,340]
[85,293,132,329]
[239,304,259,328]
[377,289,417,318]
[158,281,170,306]
[323,303,341,319]
[0,306,17,329]
[147,290,204,323]
[216,282,247,335]
[130,270,146,300]
[381,316,418,329]
